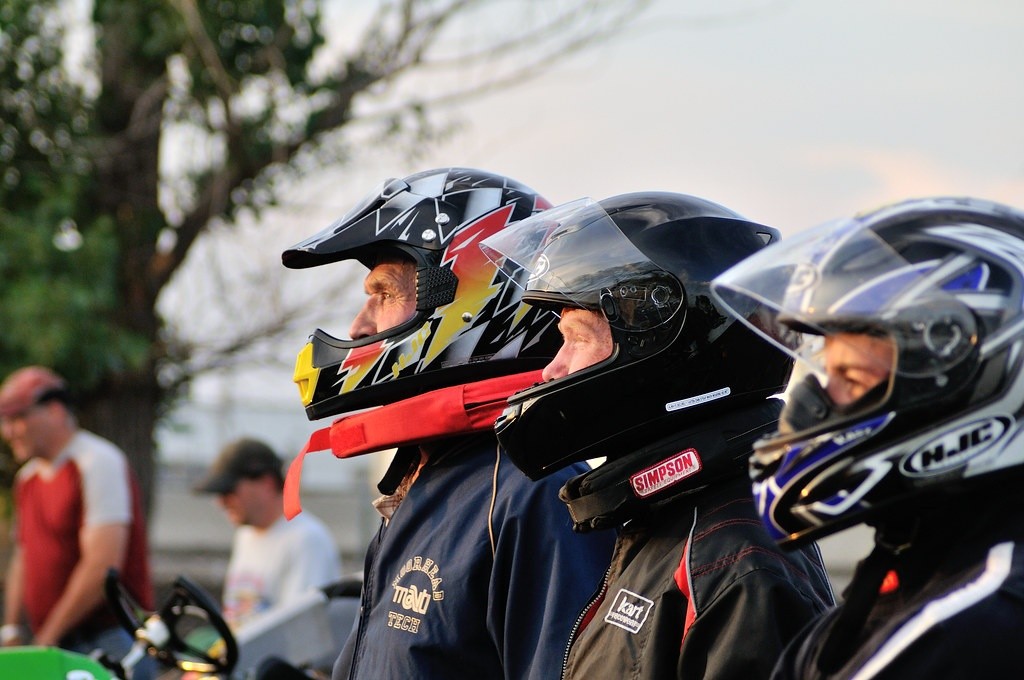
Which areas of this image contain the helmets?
[491,187,792,482]
[745,194,1024,555]
[280,161,559,461]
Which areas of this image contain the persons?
[191,437,342,680]
[281,166,618,680]
[0,364,159,680]
[477,190,838,680]
[709,197,1024,680]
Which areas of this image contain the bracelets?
[0,622,24,643]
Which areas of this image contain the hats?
[194,438,285,493]
[1,363,71,418]
